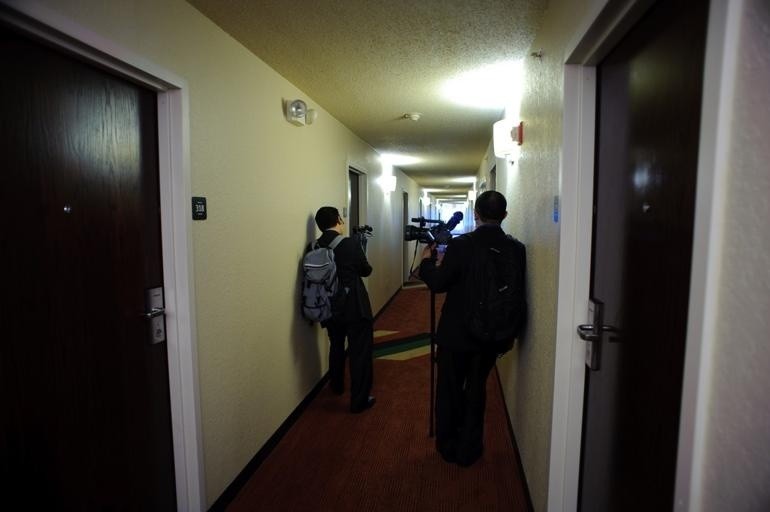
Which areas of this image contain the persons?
[419,190,528,464]
[306,207,376,413]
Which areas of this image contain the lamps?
[286,98,318,127]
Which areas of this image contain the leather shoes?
[350,395,375,413]
[329,380,344,394]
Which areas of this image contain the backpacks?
[461,231,526,350]
[302,235,350,322]
